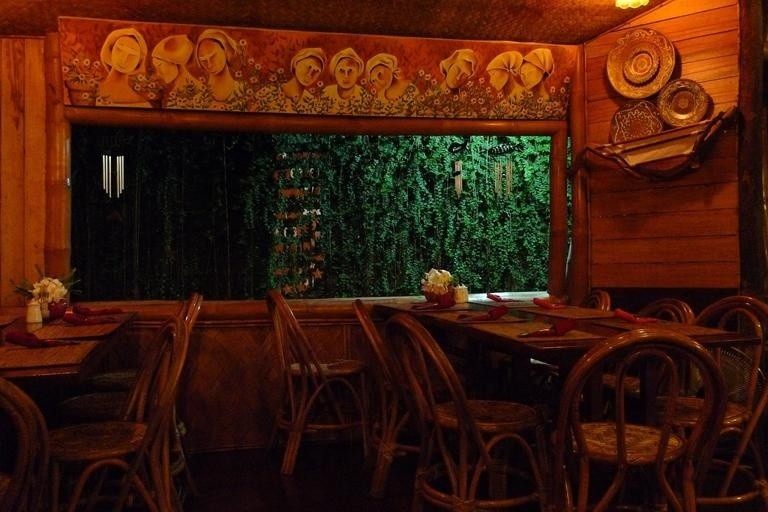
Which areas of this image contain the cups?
[454,286,468,305]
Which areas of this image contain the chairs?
[259,283,369,477]
[1,288,206,511]
[371,283,697,424]
[381,310,546,512]
[547,326,730,511]
[349,295,428,507]
[670,290,767,511]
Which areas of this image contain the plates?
[605,27,676,100]
[657,78,710,127]
[609,99,663,147]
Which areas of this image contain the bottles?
[41,301,51,324]
[27,298,43,323]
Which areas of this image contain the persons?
[194,28,238,106]
[150,34,207,109]
[520,49,556,99]
[486,51,529,104]
[366,53,419,105]
[280,46,325,100]
[94,29,152,108]
[437,49,480,93]
[321,48,369,106]
